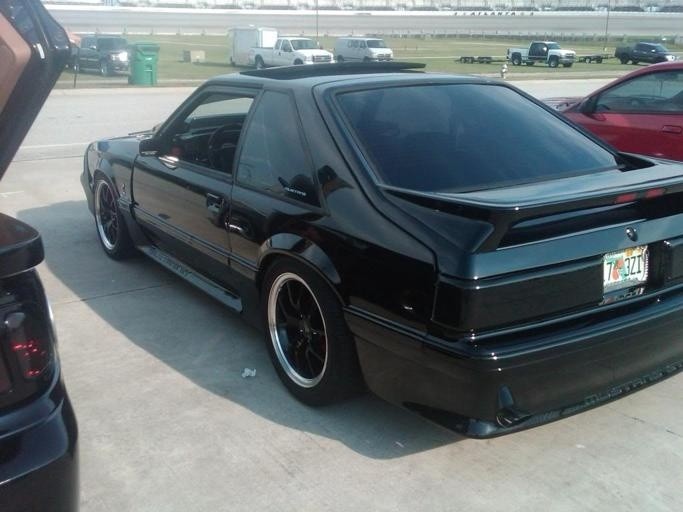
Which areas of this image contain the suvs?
[78,35,131,76]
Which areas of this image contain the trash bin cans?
[128,43,160,85]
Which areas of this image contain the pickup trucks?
[248,37,334,70]
[615,42,676,65]
[506,40,575,68]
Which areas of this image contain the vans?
[336,38,393,62]
